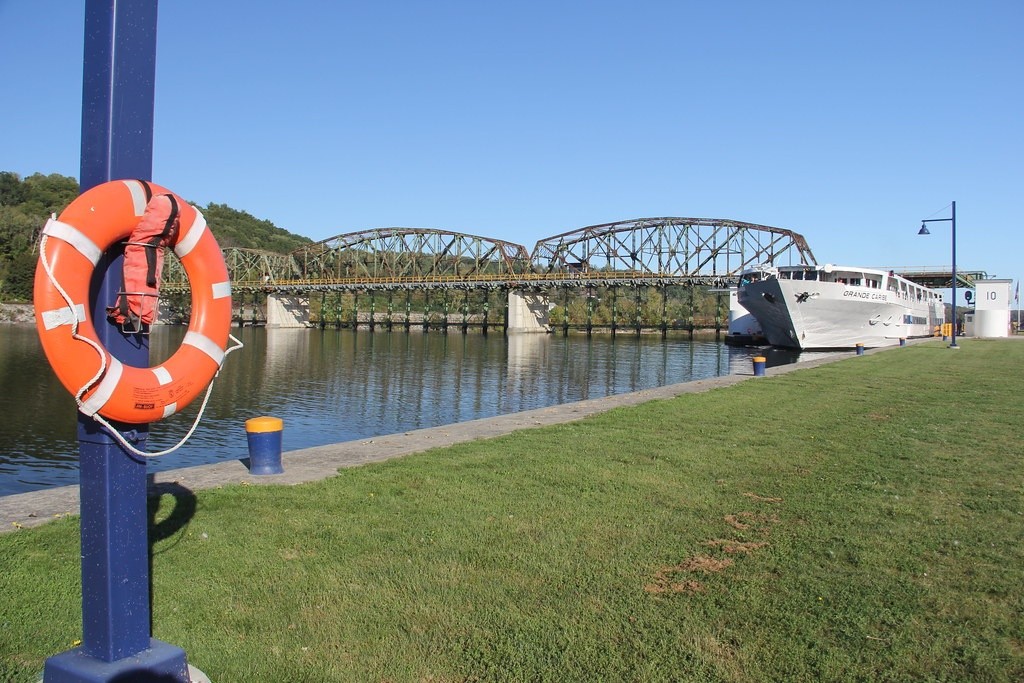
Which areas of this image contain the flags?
[1014,282,1018,304]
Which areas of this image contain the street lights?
[917,201,956,346]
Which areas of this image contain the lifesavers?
[32,178,232,424]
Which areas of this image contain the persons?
[918,293,921,301]
[956,317,962,335]
[838,277,842,283]
